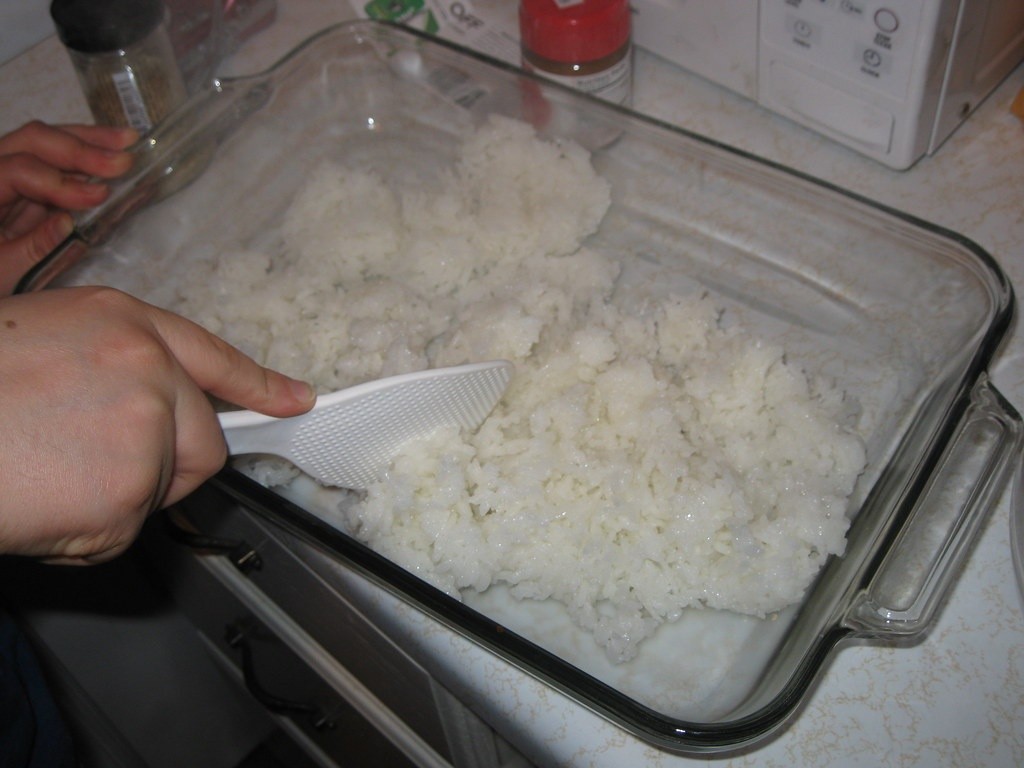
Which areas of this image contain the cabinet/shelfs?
[0,0,1024,768]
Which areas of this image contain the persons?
[0,118,319,568]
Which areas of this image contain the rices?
[158,109,867,658]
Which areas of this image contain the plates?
[10,18,1024,759]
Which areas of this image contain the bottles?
[518,0,633,152]
[48,0,215,201]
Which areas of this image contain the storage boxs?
[23,0,1024,752]
